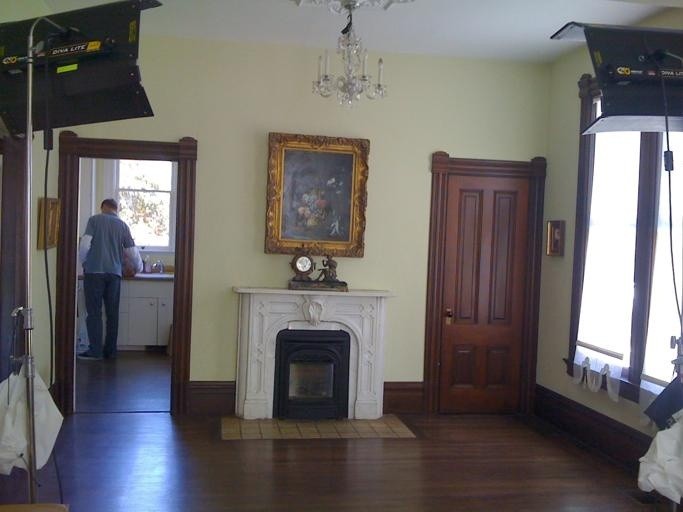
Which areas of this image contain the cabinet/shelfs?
[75,272,174,352]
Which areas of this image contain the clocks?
[286,241,350,291]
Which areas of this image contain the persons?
[76,198,143,361]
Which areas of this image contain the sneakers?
[77,352,105,361]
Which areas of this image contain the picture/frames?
[36,194,61,252]
[544,218,566,258]
[262,130,371,261]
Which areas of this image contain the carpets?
[218,410,418,443]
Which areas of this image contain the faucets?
[151,259,165,273]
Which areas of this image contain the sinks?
[134,272,174,279]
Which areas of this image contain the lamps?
[291,0,416,108]
